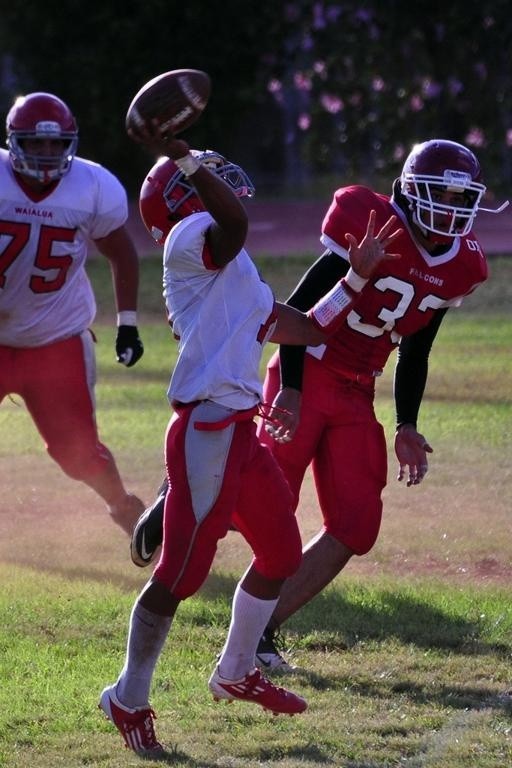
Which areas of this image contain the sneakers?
[255,633,306,676]
[98,684,163,756]
[110,475,169,567]
[209,663,307,717]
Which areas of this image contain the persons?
[0,90,157,570]
[126,137,493,679]
[97,118,404,757]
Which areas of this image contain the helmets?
[140,150,255,245]
[6,92,78,183]
[400,139,485,236]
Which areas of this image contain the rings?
[398,464,426,483]
[421,441,427,449]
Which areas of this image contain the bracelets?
[344,267,371,293]
[115,309,140,327]
[309,278,363,340]
[173,153,204,179]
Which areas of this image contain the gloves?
[115,325,143,366]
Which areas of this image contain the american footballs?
[125,68,210,142]
